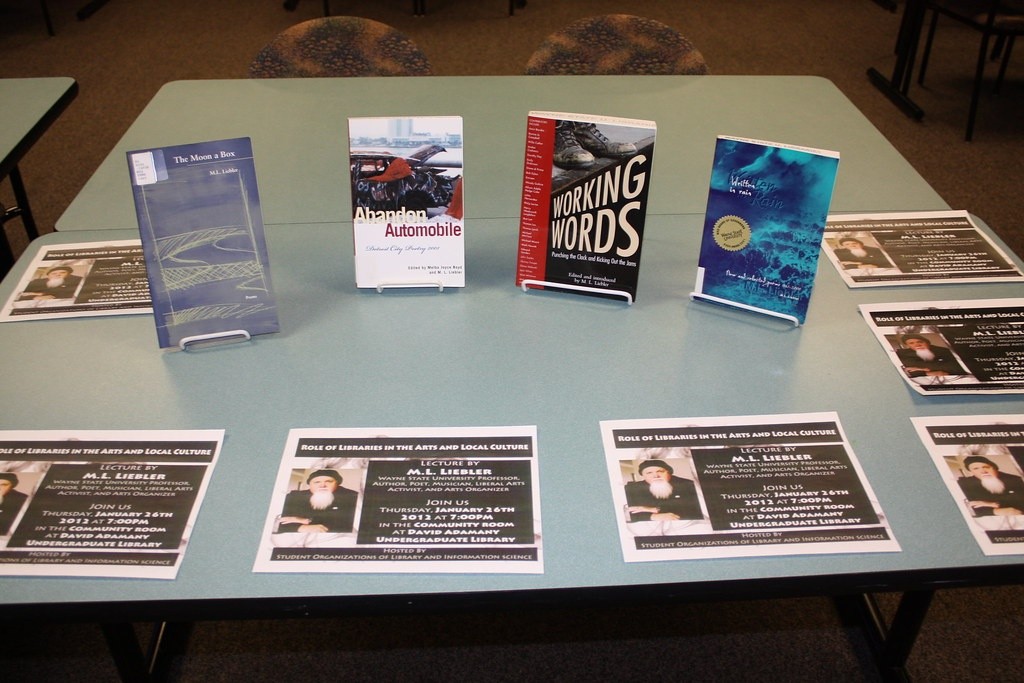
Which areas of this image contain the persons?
[896,335,968,379]
[19,266,82,299]
[958,456,1024,517]
[625,460,704,524]
[834,238,893,270]
[0,473,29,536]
[277,469,358,534]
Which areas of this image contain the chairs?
[523,14,708,75]
[253,18,436,77]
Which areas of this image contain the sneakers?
[572,121,638,155]
[553,120,595,167]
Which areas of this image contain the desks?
[0,75,1024,683]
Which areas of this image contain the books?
[696,136,841,328]
[346,116,466,288]
[516,111,657,303]
[126,136,280,347]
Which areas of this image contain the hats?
[839,238,863,246]
[47,267,73,275]
[307,470,343,485]
[0,472,18,489]
[964,456,998,471]
[902,333,931,345]
[639,460,673,476]
